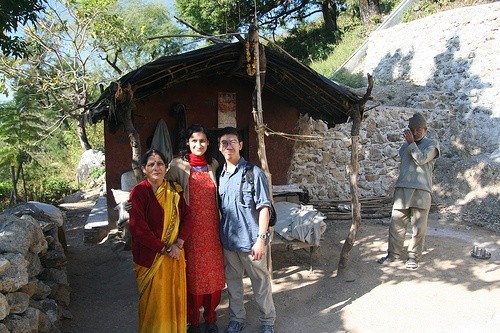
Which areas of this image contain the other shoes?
[206,325,218,333]
[379,256,392,264]
[188,325,200,333]
[407,258,417,267]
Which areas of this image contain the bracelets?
[175,241,183,250]
[165,247,171,254]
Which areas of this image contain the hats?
[408,113,427,128]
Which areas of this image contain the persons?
[215,126,277,333]
[124,123,226,333]
[377,113,440,268]
[128,149,194,333]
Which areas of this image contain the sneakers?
[262,325,273,333]
[226,321,245,333]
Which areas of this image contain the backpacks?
[216,163,277,226]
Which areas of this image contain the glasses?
[220,141,240,146]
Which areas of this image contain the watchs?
[256,233,267,240]
